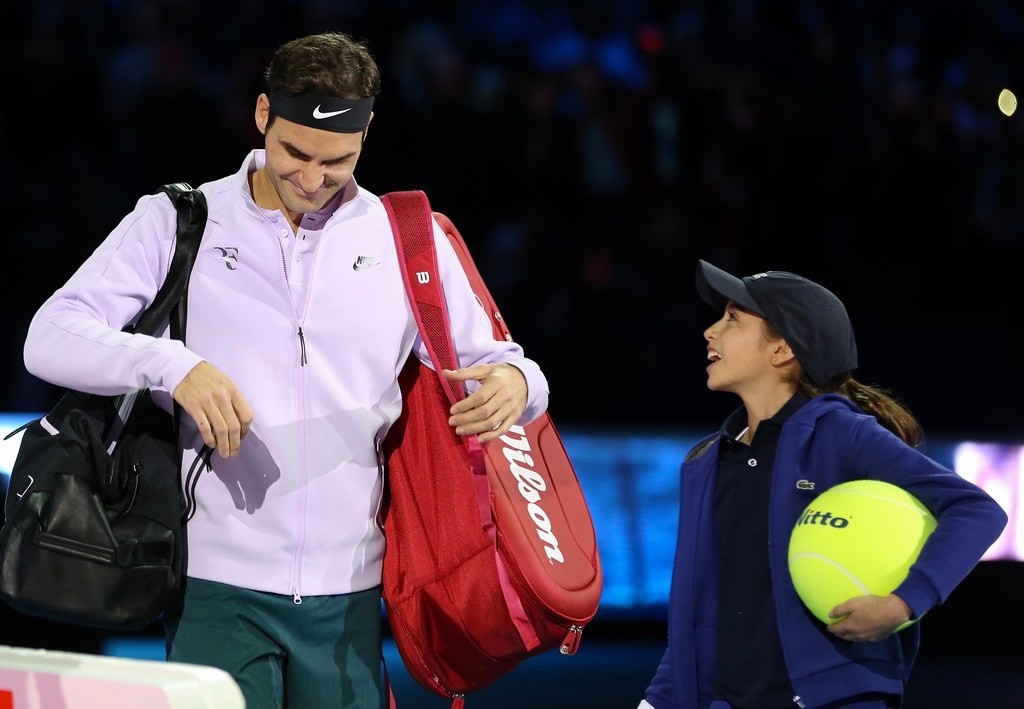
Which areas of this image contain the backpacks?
[0,181,210,644]
[378,191,600,709]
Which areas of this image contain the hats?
[697,256,858,391]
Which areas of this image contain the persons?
[640,261,1008,709]
[22,30,549,709]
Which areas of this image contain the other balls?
[787,479,939,639]
